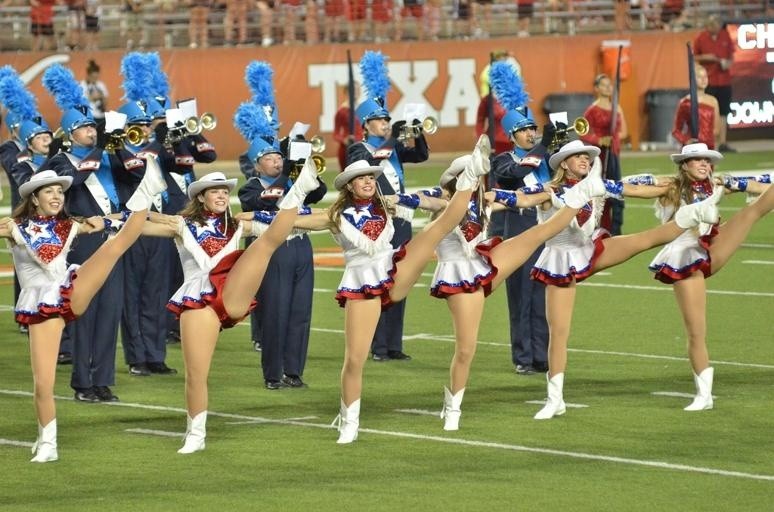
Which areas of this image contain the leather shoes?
[165,330,177,344]
[388,351,411,360]
[94,387,119,402]
[280,373,308,391]
[515,364,534,375]
[18,323,28,334]
[373,354,389,361]
[264,380,285,389]
[254,342,263,352]
[58,352,73,364]
[128,363,150,376]
[75,388,100,403]
[146,362,177,375]
[533,364,549,372]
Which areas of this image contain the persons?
[490,125,569,378]
[1,1,773,381]
[713,173,774,195]
[618,141,773,414]
[36,115,147,404]
[345,115,440,361]
[480,139,681,420]
[233,131,493,445]
[0,156,180,463]
[235,150,326,393]
[379,153,607,432]
[81,154,320,456]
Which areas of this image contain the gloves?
[282,160,295,176]
[280,137,289,156]
[155,122,168,144]
[297,158,306,173]
[391,120,406,138]
[296,134,305,141]
[555,121,568,140]
[111,129,125,145]
[95,120,111,149]
[540,122,555,146]
[412,119,423,134]
[172,120,186,137]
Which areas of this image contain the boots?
[456,134,491,191]
[560,156,606,209]
[675,185,725,228]
[278,156,320,215]
[126,154,168,213]
[440,385,466,431]
[30,417,58,463]
[331,398,361,444]
[684,366,714,412]
[534,371,566,420]
[177,410,208,454]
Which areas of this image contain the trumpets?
[164,116,202,145]
[384,117,439,142]
[309,135,326,153]
[105,126,145,155]
[200,112,217,130]
[274,155,326,180]
[528,117,589,154]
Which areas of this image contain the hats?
[187,172,238,201]
[61,105,97,136]
[334,160,383,190]
[440,154,472,188]
[500,105,537,138]
[19,170,74,200]
[117,100,152,122]
[262,103,279,130]
[360,98,391,128]
[246,133,283,167]
[5,110,21,132]
[670,143,724,164]
[17,110,53,143]
[145,99,171,119]
[549,140,601,172]
